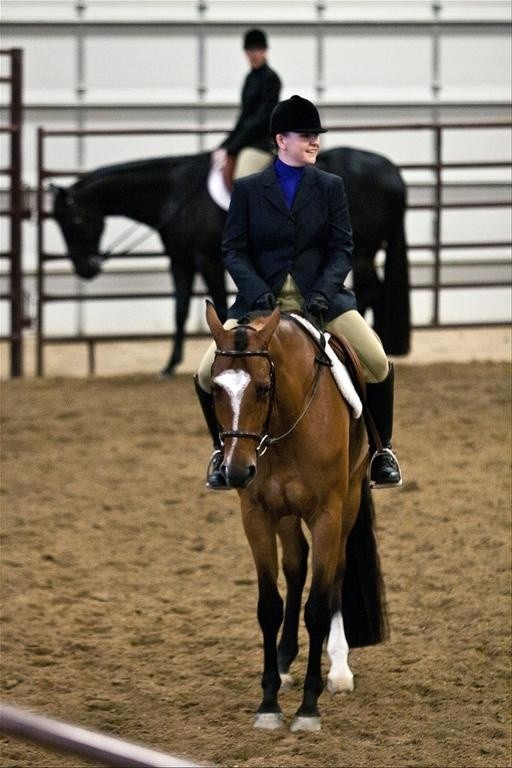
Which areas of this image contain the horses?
[50,144,412,381]
[202,298,392,735]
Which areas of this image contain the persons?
[215,27,283,199]
[195,93,404,491]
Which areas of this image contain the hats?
[244,30,267,49]
[269,95,329,138]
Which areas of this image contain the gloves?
[253,291,277,313]
[304,290,332,315]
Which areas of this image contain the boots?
[362,360,401,484]
[192,373,233,490]
